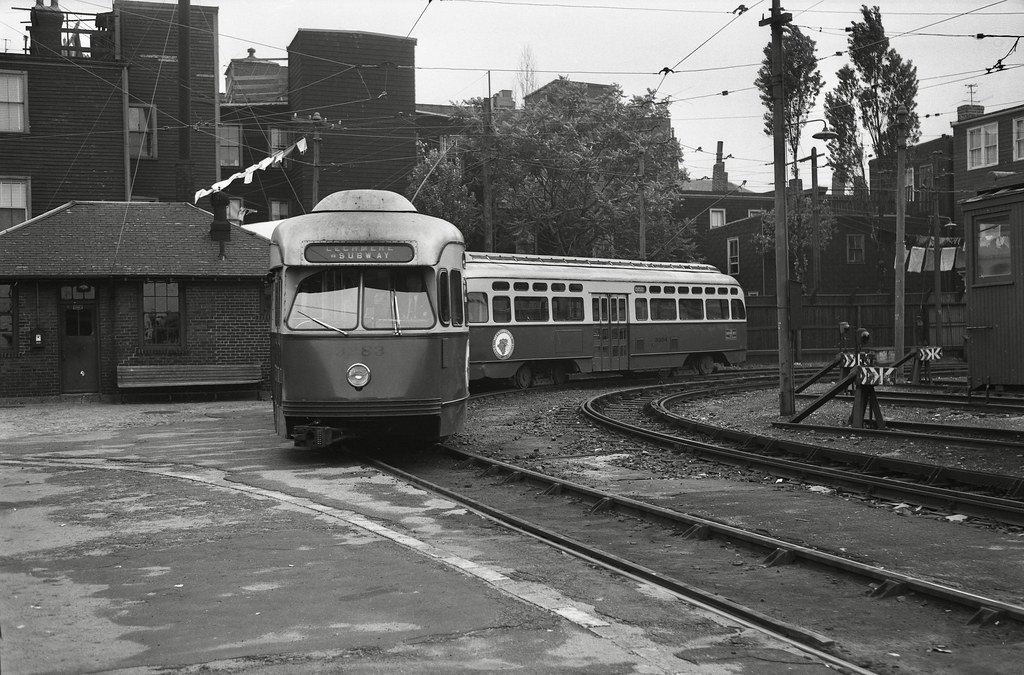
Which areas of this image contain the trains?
[270,189,748,449]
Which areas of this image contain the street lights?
[774,119,838,418]
[932,215,957,358]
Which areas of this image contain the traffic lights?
[840,322,850,335]
[856,328,870,346]
